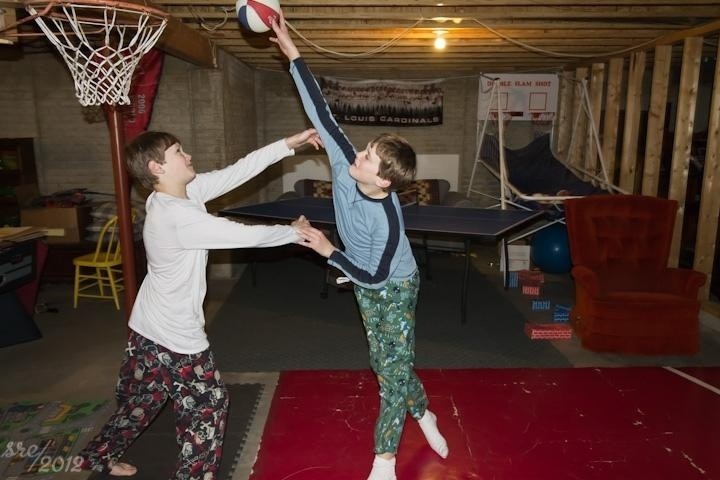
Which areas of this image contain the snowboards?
[236,0,280,34]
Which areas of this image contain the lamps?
[563,195,709,357]
[71,207,139,311]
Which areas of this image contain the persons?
[266,6,454,480]
[67,126,326,480]
[323,85,445,117]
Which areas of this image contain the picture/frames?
[217,195,546,326]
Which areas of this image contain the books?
[18,201,94,245]
[499,237,532,274]
